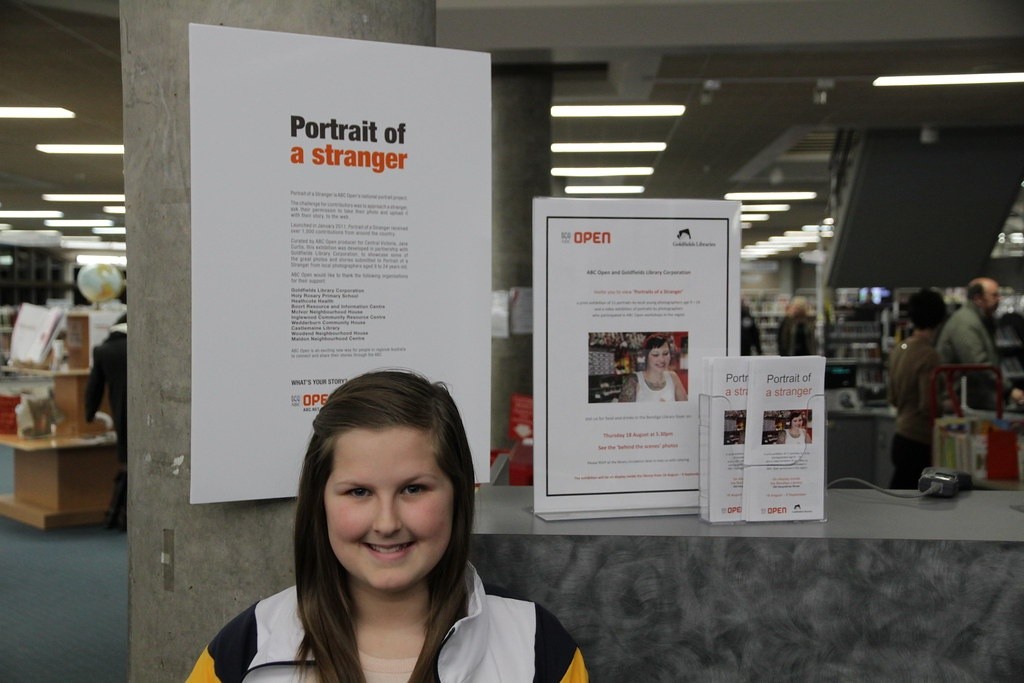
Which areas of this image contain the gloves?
[86,409,99,423]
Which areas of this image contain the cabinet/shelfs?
[0,368,119,529]
[743,287,1023,409]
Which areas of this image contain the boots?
[102,471,127,530]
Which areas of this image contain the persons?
[83,313,127,535]
[777,412,812,444]
[936,277,1024,415]
[183,369,595,683]
[886,287,952,493]
[740,299,764,357]
[840,291,881,322]
[775,294,817,358]
[617,334,687,402]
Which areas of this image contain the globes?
[77,262,123,310]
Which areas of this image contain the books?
[8,303,65,364]
